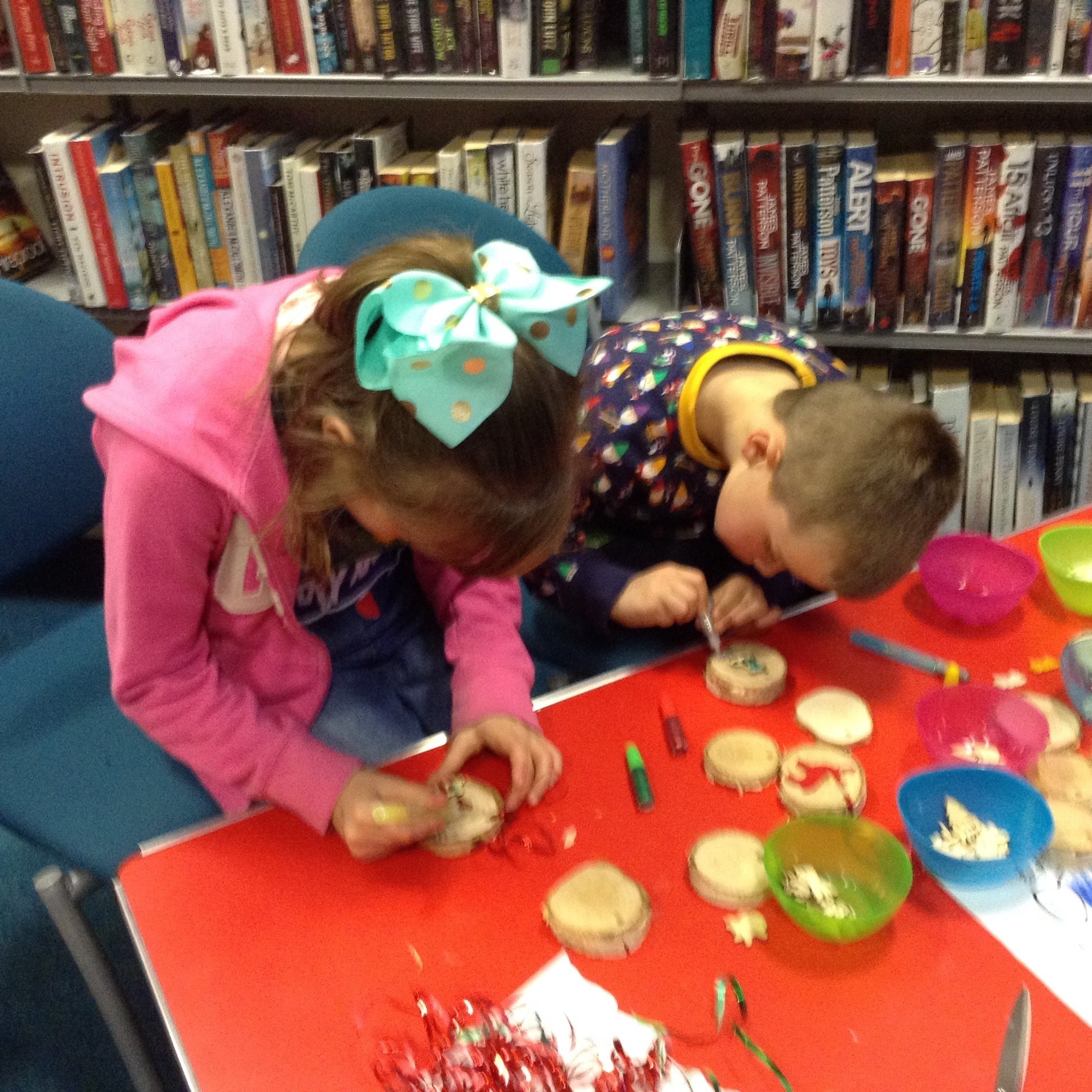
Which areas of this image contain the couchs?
[3,281,221,1092]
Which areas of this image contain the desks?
[107,505,1092,1092]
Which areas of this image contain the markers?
[372,797,457,832]
[850,629,972,685]
[701,600,726,659]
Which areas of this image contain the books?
[0,1,1091,568]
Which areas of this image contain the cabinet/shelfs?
[1,0,1091,551]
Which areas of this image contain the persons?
[537,297,973,676]
[81,234,594,862]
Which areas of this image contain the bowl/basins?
[897,762,1054,887]
[913,683,1050,776]
[760,812,914,946]
[1057,629,1091,723]
[1038,525,1091,617]
[920,533,1039,624]
